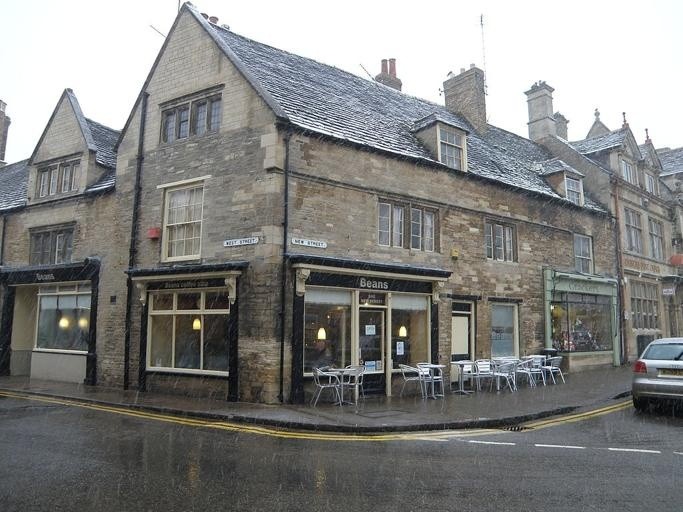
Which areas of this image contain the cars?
[630,337,683,410]
[360,332,409,360]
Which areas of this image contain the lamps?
[190,316,201,330]
[317,310,326,339]
[58,311,87,329]
[398,311,407,337]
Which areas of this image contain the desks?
[450,362,478,396]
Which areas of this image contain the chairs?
[458,354,566,394]
[308,364,367,409]
[397,363,445,401]
